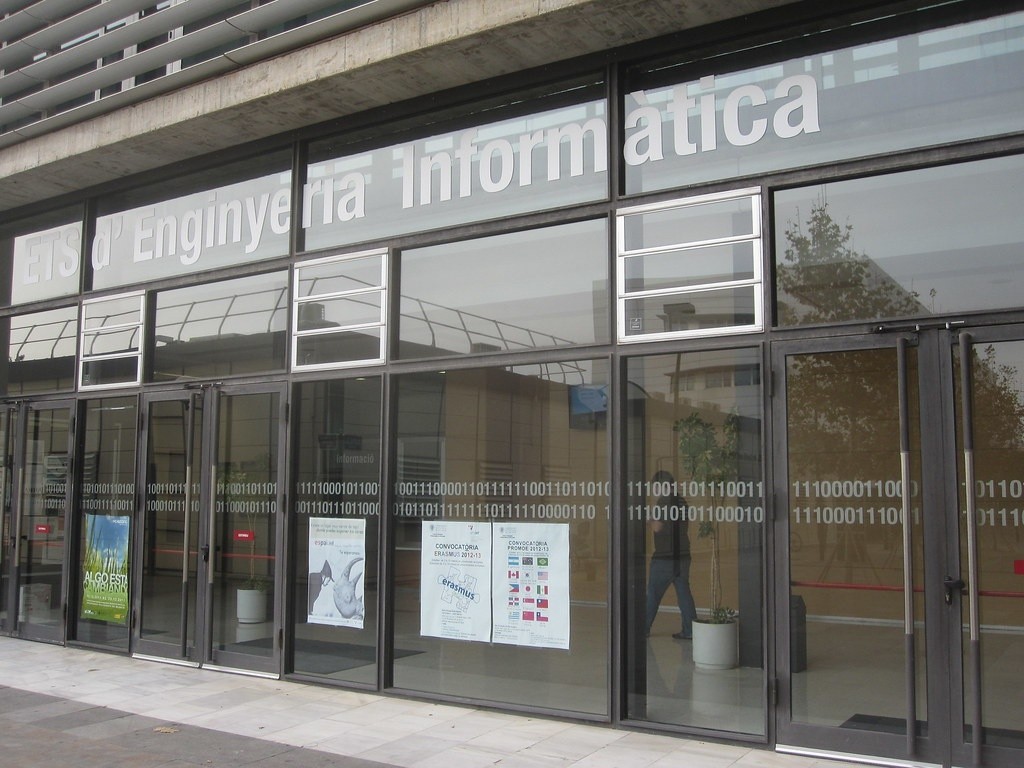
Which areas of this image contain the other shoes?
[645,628,651,637]
[673,633,693,638]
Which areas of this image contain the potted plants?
[672,402,740,669]
[219,450,271,624]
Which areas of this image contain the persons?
[646,470,697,640]
